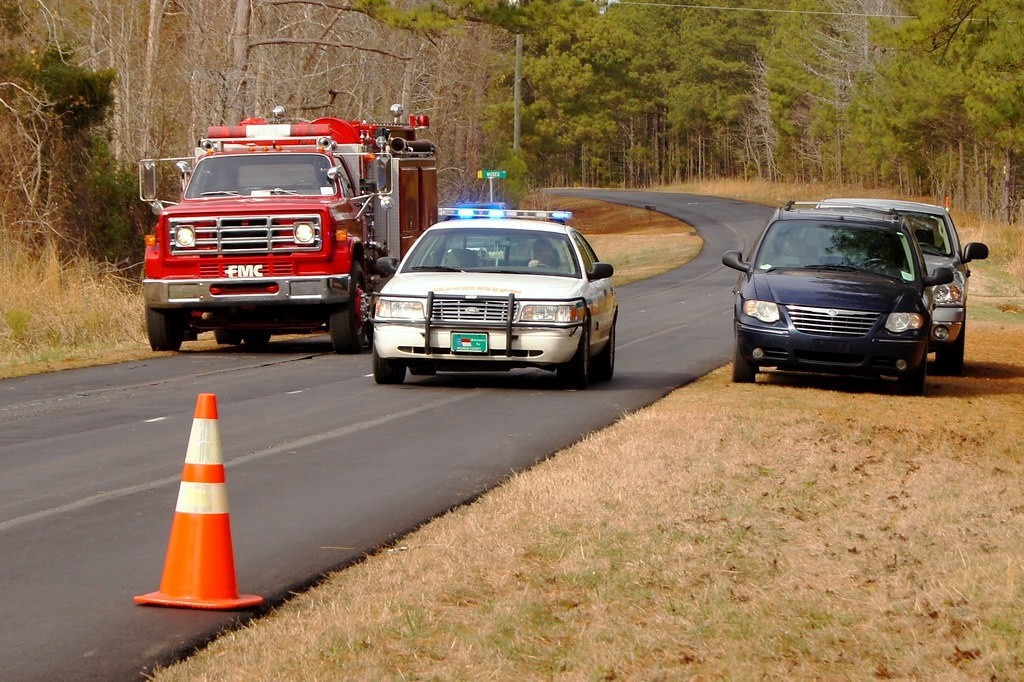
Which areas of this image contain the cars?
[364,204,620,392]
[722,199,953,397]
[816,195,990,376]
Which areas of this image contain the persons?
[524,238,570,274]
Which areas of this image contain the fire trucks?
[137,102,439,356]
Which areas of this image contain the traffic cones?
[131,392,264,612]
